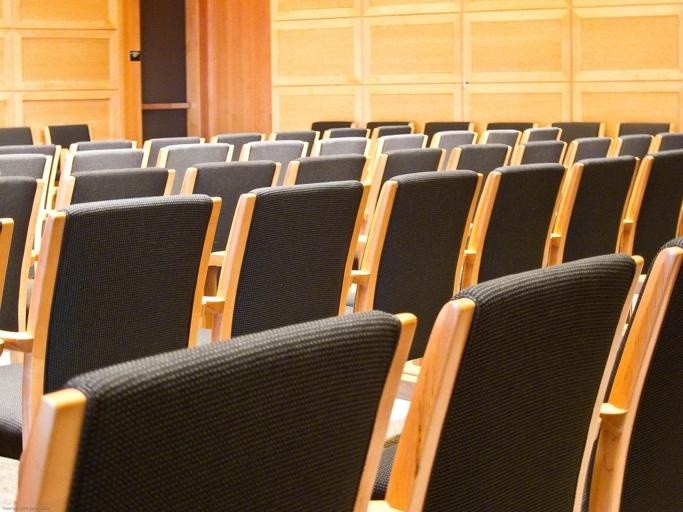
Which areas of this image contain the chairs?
[619,149,682,274]
[13,310,418,512]
[552,122,601,146]
[547,155,639,272]
[369,249,647,511]
[615,122,670,141]
[563,137,613,167]
[654,132,682,154]
[1,192,224,468]
[615,133,654,160]
[1,122,569,365]
[583,233,680,511]
